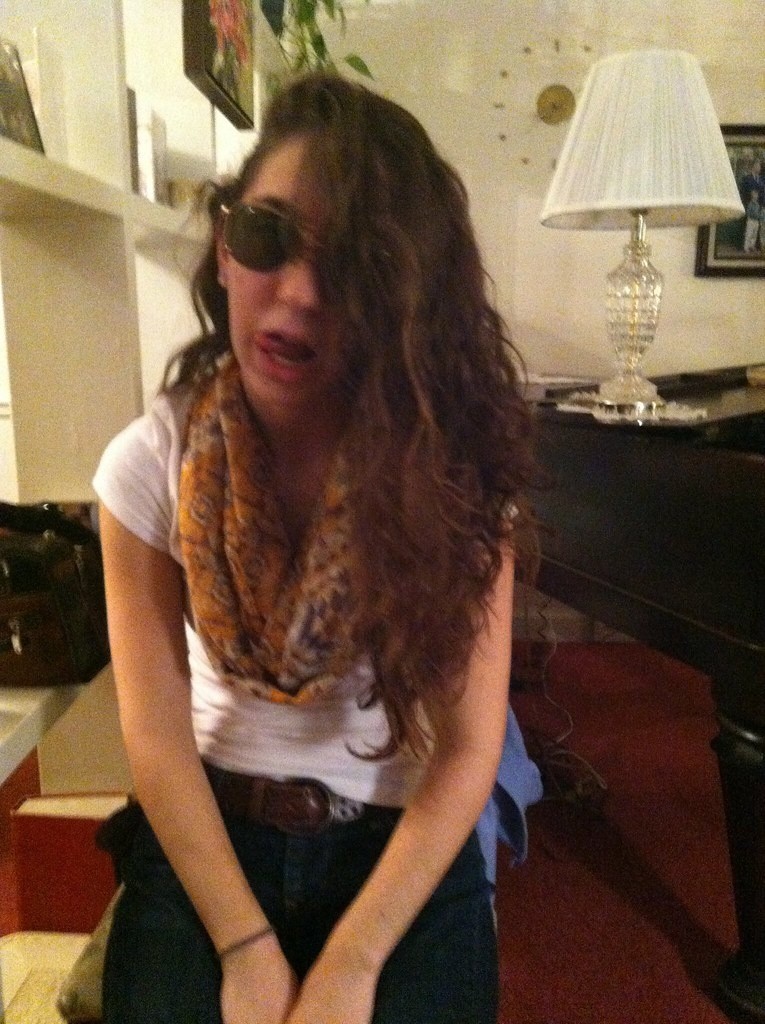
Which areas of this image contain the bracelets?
[217,927,274,960]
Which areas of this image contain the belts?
[210,774,366,838]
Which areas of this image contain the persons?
[740,160,765,254]
[91,72,536,1024]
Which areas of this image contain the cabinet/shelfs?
[0,0,295,1024]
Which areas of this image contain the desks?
[512,363,765,1024]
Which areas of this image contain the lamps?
[541,51,746,422]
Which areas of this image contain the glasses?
[220,198,307,273]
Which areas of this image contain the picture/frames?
[184,0,256,131]
[695,125,765,276]
